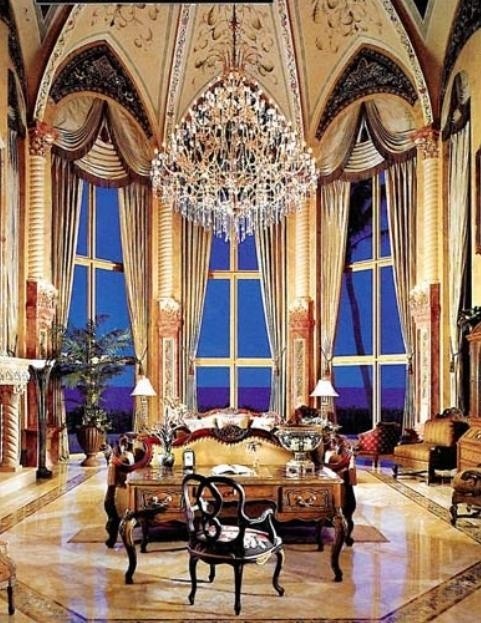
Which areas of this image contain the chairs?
[450,464,481,528]
[180,472,286,616]
[352,407,464,486]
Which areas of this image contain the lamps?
[309,377,340,423]
[128,375,158,434]
[148,4,322,247]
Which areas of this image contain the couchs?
[104,422,358,556]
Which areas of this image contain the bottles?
[182,442,196,475]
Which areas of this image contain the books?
[211,463,254,475]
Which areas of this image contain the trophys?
[274,430,326,478]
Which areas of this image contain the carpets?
[67,524,389,545]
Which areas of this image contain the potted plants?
[45,312,138,468]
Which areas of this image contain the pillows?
[184,413,277,435]
